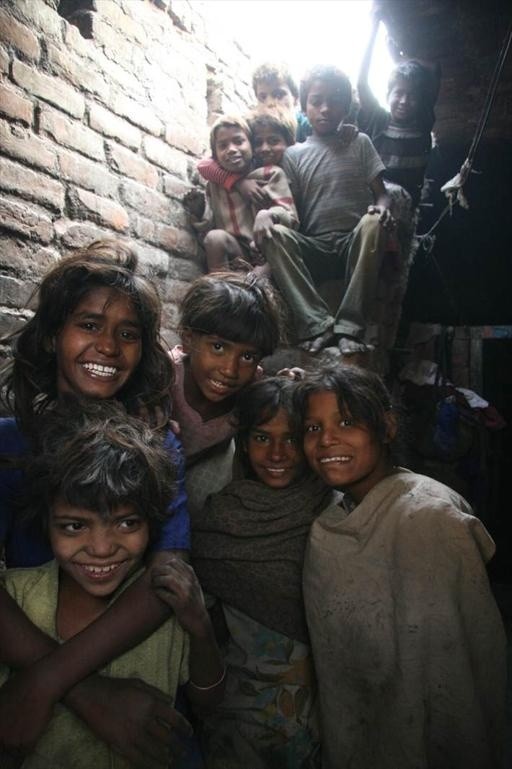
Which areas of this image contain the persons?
[191,376,334,769]
[165,272,302,513]
[1,238,194,769]
[182,3,442,356]
[1,399,227,769]
[292,359,511,769]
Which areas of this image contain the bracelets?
[187,664,227,691]
